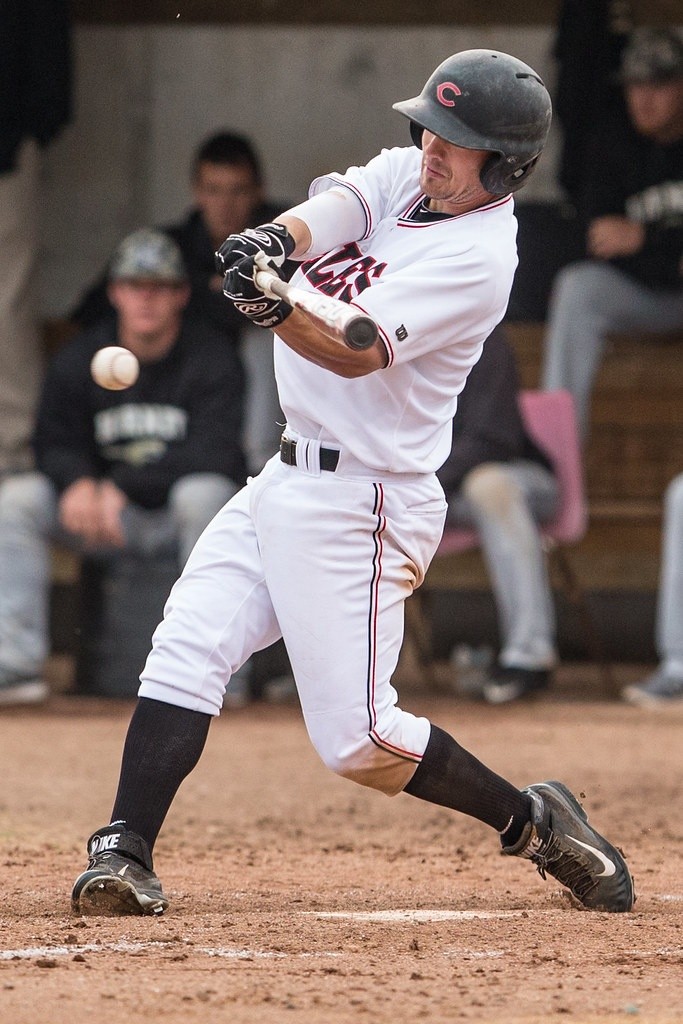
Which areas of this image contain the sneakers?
[71,820,169,917]
[500,781,633,914]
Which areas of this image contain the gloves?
[221,250,295,329]
[213,223,297,280]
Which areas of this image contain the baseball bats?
[255,272,378,354]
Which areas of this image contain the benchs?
[424,328,683,590]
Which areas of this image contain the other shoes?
[224,661,299,708]
[0,675,53,707]
[621,669,683,715]
[479,666,549,705]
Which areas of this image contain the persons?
[1,1,294,707]
[72,49,638,917]
[437,30,682,706]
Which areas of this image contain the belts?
[280,436,340,472]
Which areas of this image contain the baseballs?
[90,345,140,391]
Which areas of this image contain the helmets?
[392,49,552,195]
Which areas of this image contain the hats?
[105,228,190,289]
[614,26,683,84]
